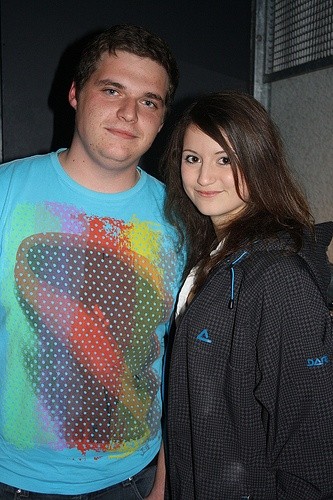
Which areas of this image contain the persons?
[162,91,333,500]
[0,27,190,499]
